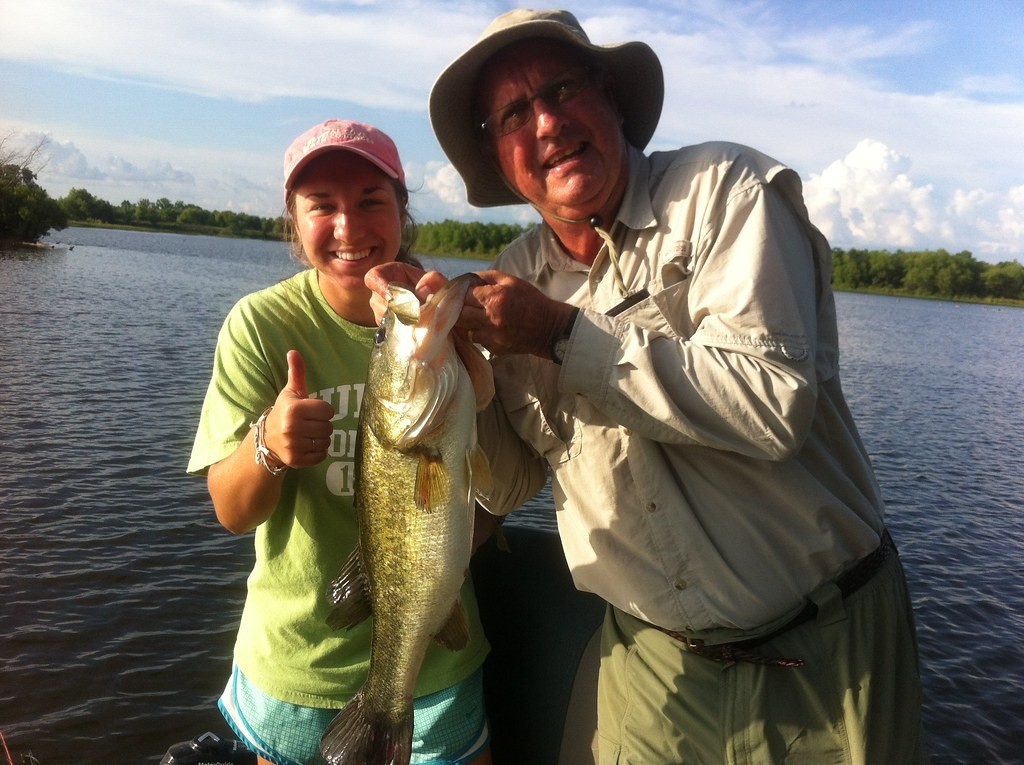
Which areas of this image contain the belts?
[635,537,891,666]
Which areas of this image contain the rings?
[467,328,474,343]
[311,439,318,452]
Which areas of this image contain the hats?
[428,7,665,207]
[283,119,406,201]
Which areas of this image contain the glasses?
[480,66,597,138]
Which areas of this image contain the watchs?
[552,306,580,367]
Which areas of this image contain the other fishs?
[321,272,495,765]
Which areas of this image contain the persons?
[183,118,494,765]
[362,8,922,765]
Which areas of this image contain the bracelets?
[247,406,290,478]
